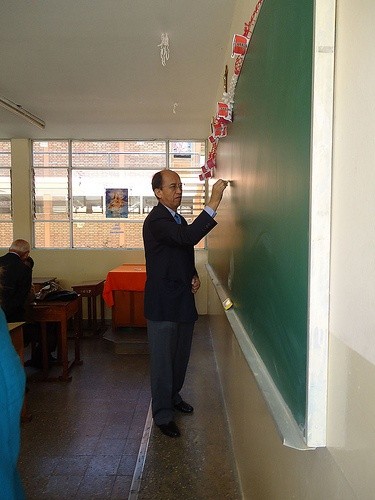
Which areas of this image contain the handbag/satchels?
[44,290,78,303]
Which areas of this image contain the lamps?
[0,94,46,130]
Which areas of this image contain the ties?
[175,214,180,224]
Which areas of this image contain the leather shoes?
[175,401,193,413]
[158,421,179,438]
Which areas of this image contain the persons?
[21,256,37,305]
[0,238,63,371]
[141,169,229,438]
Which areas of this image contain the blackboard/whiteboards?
[202,0,335,452]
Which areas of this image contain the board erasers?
[222,298,233,311]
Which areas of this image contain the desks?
[31,294,82,381]
[32,277,59,296]
[7,322,27,367]
[103,263,147,331]
[71,280,105,339]
